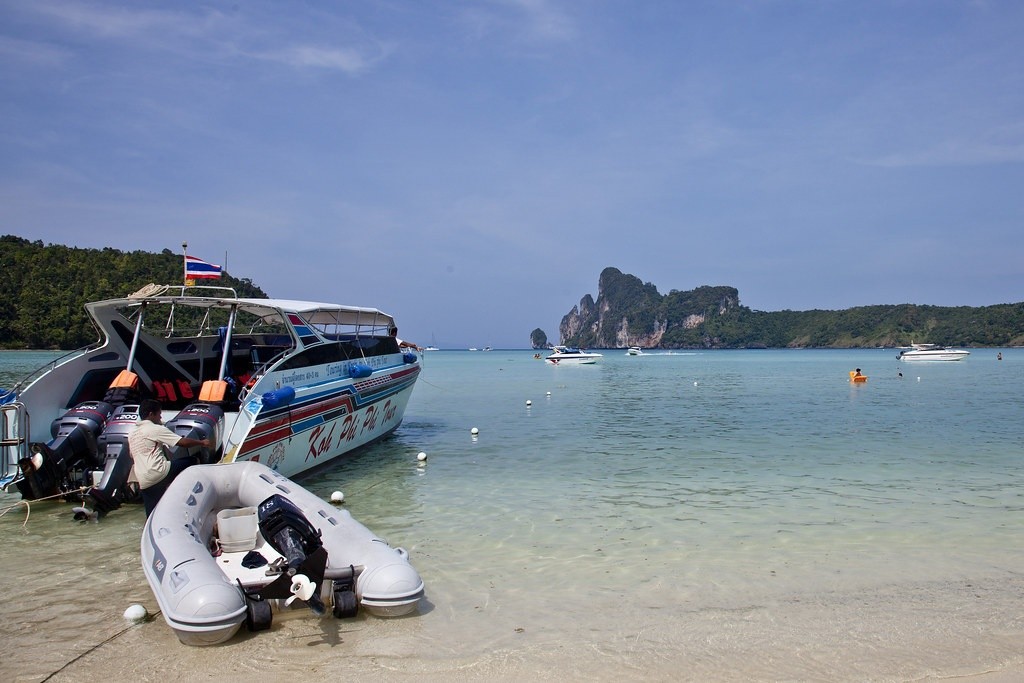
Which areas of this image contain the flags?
[186,256,222,280]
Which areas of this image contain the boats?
[0,240,422,523]
[469,348,477,351]
[897,338,970,361]
[483,346,493,351]
[549,345,566,350]
[533,354,542,359]
[137,461,427,653]
[628,347,642,355]
[849,370,867,382]
[547,344,604,366]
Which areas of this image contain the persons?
[127,401,212,521]
[387,326,424,351]
[853,369,862,377]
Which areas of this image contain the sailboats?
[424,330,440,350]
